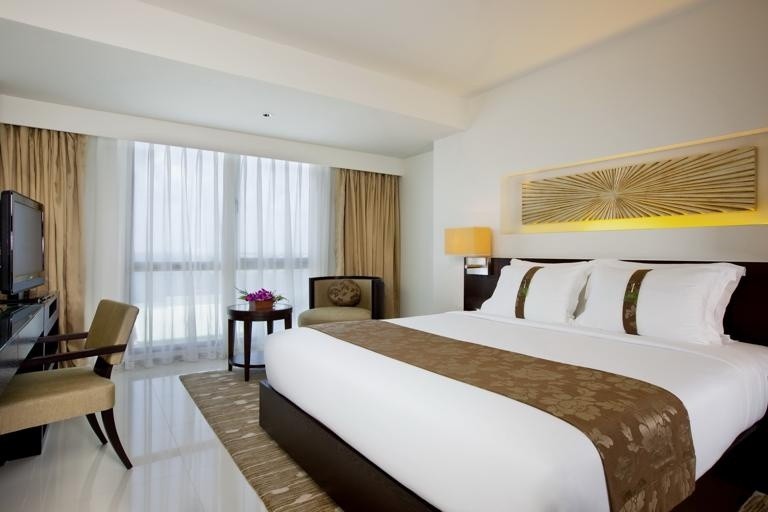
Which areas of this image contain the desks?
[227,302,294,380]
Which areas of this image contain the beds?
[259,256,768,512]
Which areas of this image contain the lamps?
[441,226,493,276]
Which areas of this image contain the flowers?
[235,285,285,302]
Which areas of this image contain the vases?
[248,299,273,310]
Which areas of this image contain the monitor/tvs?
[0,190,55,303]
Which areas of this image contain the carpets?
[180,368,344,512]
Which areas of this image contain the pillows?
[328,279,362,307]
[474,256,747,348]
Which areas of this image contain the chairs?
[0,299,141,469]
[298,275,384,326]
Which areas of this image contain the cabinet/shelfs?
[1,290,64,458]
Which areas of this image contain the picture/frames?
[497,125,768,234]
